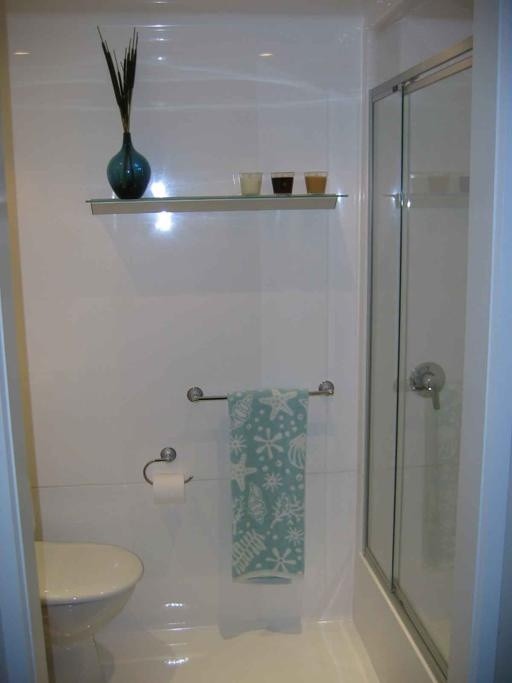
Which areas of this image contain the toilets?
[32,539,143,683]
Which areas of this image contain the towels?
[226,388,309,583]
[422,382,461,568]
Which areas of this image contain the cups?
[239,172,328,195]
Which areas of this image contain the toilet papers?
[152,474,186,507]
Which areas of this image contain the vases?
[107,132,150,200]
